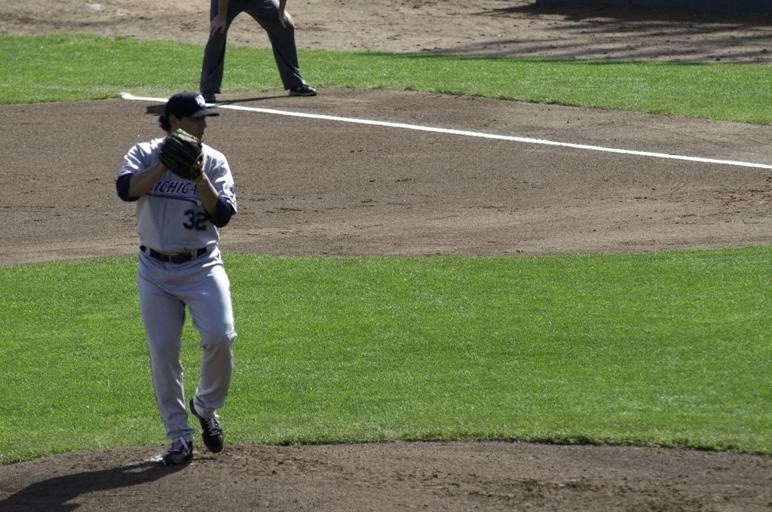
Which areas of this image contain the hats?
[165,92,219,119]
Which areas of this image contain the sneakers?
[163,439,193,465]
[289,84,317,96]
[203,90,216,102]
[190,399,223,452]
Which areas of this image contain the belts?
[140,245,206,263]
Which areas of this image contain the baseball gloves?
[157,128,203,182]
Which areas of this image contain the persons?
[199,0,316,100]
[113,90,237,467]
